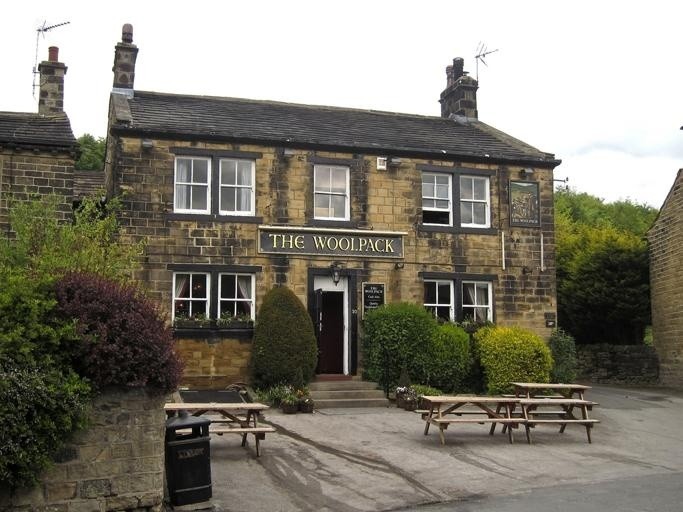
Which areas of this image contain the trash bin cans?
[166,416,212,511]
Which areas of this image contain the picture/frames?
[509,178,542,228]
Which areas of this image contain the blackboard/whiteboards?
[362,282,386,320]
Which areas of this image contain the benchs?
[414,394,603,424]
[165,413,258,423]
[163,425,278,433]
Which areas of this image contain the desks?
[419,395,521,446]
[508,381,593,428]
[518,397,600,445]
[163,402,270,457]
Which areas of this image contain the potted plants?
[265,383,314,413]
[396,384,443,411]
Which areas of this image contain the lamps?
[142,140,154,148]
[518,168,534,176]
[329,262,344,286]
[384,156,401,168]
[279,149,294,159]
